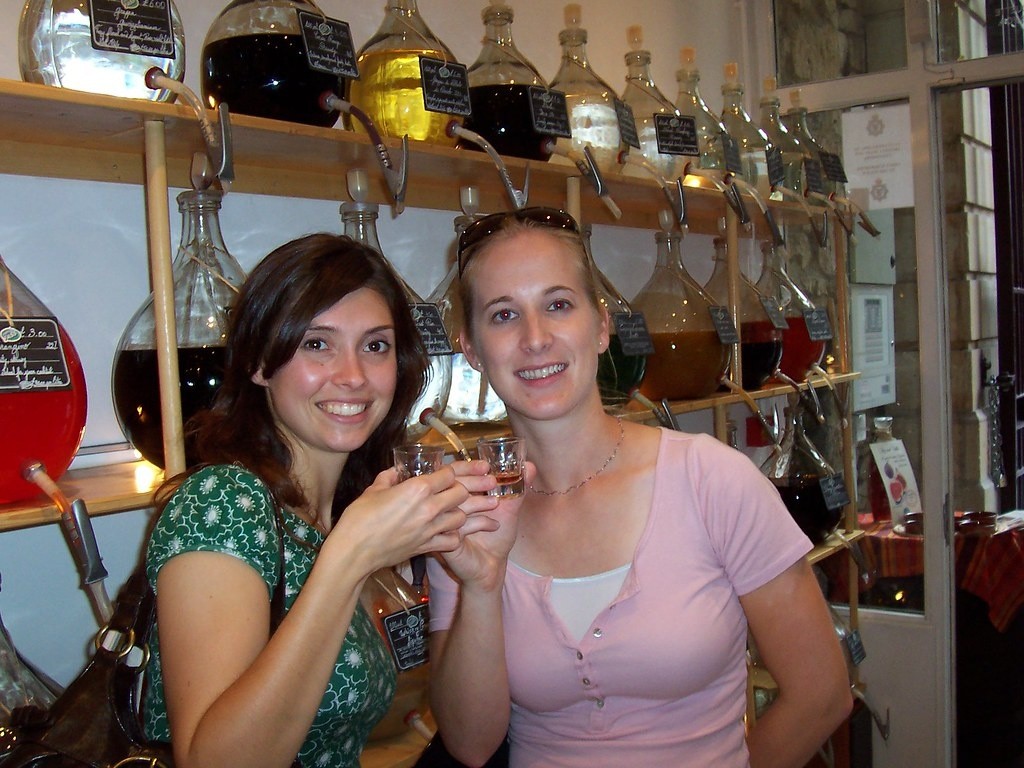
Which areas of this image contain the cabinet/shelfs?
[0,78,864,768]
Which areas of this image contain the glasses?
[459,206,579,280]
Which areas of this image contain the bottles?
[2,0,894,767]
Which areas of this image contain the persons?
[140,233,469,768]
[425,205,853,768]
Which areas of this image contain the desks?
[821,510,1024,702]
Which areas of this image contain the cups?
[390,444,446,484]
[476,435,527,499]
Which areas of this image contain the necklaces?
[305,508,328,534]
[529,418,624,496]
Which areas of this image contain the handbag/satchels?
[0,459,285,768]
